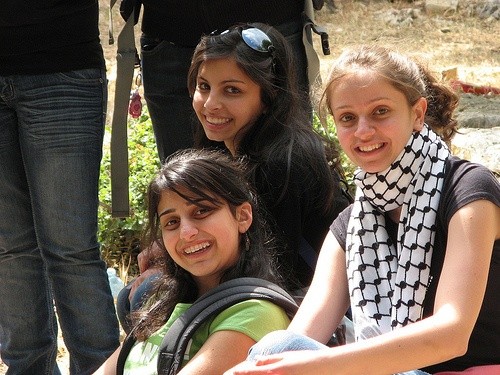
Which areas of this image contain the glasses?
[208,23,277,73]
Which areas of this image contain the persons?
[187,23,351,291]
[89,146,294,375]
[119,0,314,163]
[221,42,500,375]
[0,0,121,375]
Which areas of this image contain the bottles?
[107,268,127,338]
